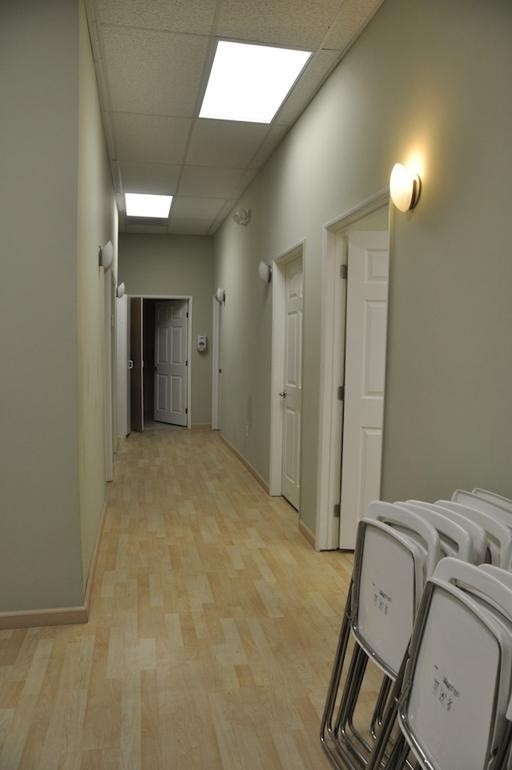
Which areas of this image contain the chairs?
[373,558,511,769]
[319,488,512,770]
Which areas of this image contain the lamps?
[118,283,125,298]
[389,163,421,212]
[102,241,113,268]
[215,288,225,302]
[258,261,272,283]
